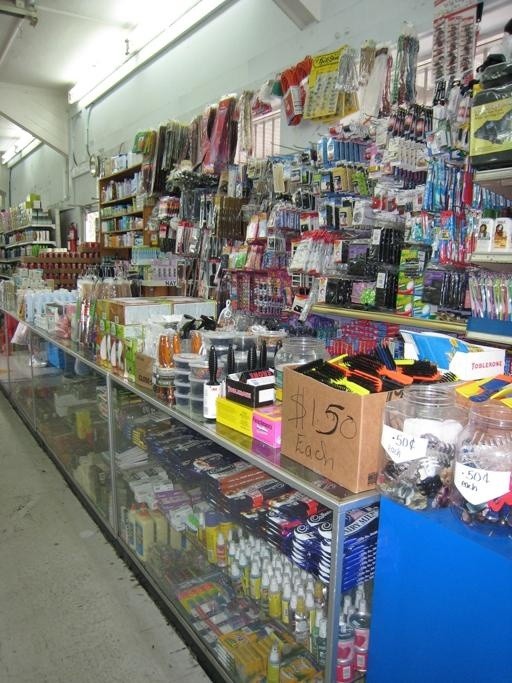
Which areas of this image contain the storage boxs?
[279,363,401,492]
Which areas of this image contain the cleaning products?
[126,500,194,562]
[19,284,72,320]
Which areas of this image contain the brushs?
[293,342,459,396]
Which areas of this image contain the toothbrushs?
[423,159,512,322]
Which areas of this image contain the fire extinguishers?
[66,222,79,252]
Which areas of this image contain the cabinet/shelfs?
[0,208,60,279]
[462,154,511,351]
[25,254,102,293]
[97,160,146,248]
[2,313,376,682]
